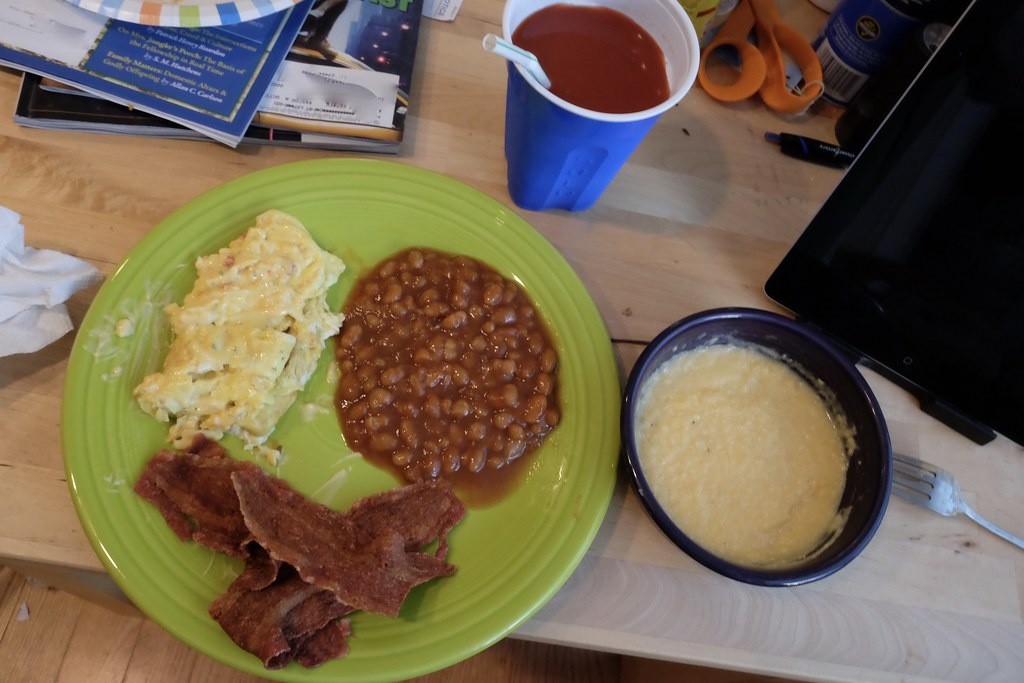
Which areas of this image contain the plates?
[61,158,624,680]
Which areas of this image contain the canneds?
[806,0,954,150]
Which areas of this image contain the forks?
[889,452,1023,552]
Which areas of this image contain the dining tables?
[0,0,1024,683]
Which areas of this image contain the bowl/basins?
[620,307,894,587]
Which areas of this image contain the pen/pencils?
[764,130,856,170]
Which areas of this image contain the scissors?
[697,0,824,114]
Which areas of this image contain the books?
[0,0,424,154]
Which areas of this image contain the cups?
[503,1,701,207]
